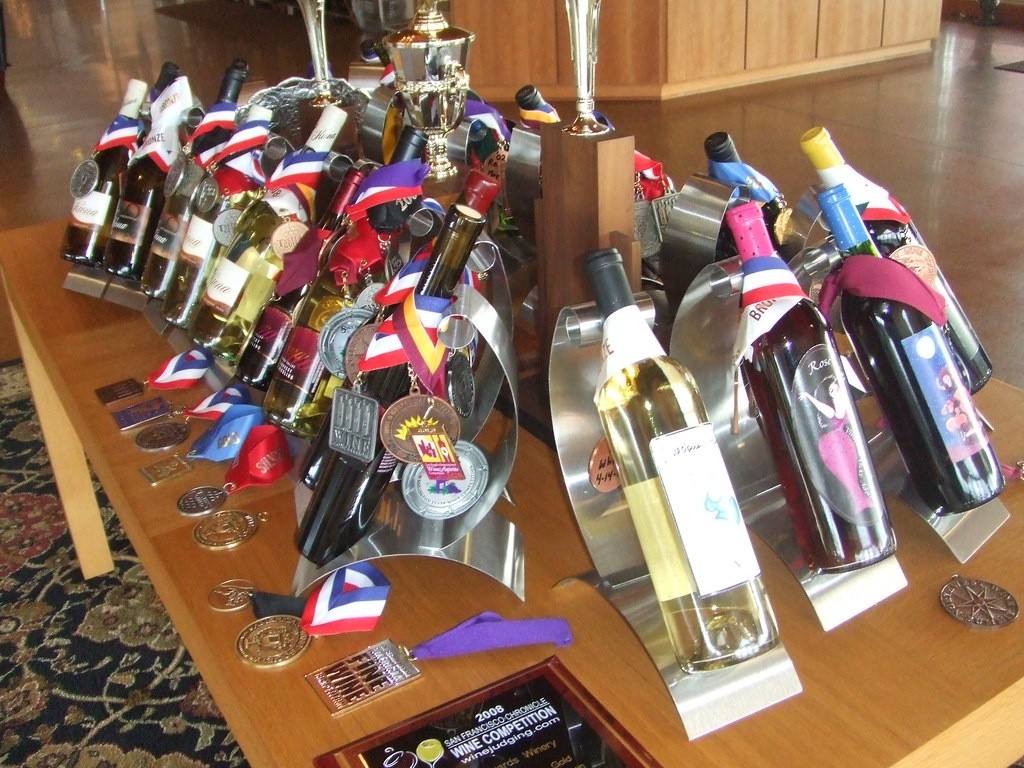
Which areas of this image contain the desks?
[0,216,1024,768]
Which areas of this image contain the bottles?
[583,246,781,670]
[799,124,993,395]
[818,184,1004,517]
[60,59,560,565]
[704,130,792,260]
[726,201,898,573]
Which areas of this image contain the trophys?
[297,0,342,110]
[565,0,611,138]
[380,0,475,182]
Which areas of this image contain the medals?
[67,147,1018,720]
